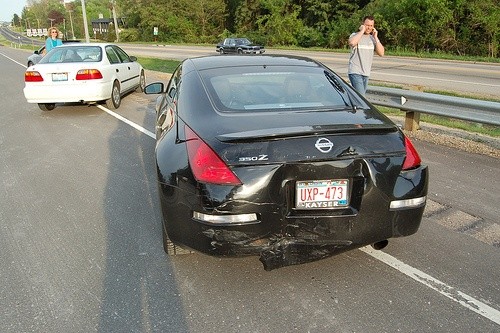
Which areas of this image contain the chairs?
[280,77,315,102]
[211,79,243,106]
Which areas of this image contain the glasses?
[52,32,57,33]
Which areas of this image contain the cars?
[216,38,265,55]
[23,42,145,112]
[143,54,429,272]
[27,40,81,68]
